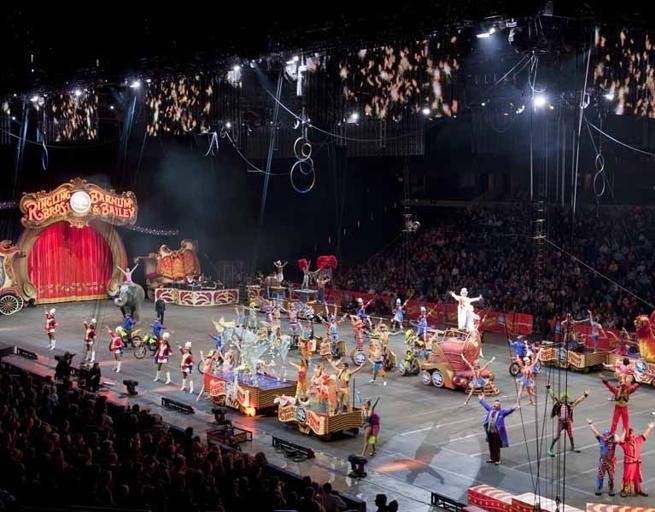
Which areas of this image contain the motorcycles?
[109,329,142,353]
[133,333,161,358]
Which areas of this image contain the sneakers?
[486,459,501,464]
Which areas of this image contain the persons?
[0,203,655,511]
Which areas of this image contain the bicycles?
[198,349,223,375]
[509,357,541,375]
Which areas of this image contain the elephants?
[113,283,146,321]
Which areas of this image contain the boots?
[112,361,120,372]
[153,371,161,381]
[189,381,193,394]
[180,379,186,390]
[570,438,581,452]
[47,340,55,350]
[85,352,95,362]
[165,372,171,384]
[547,440,557,456]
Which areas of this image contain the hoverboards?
[399,358,421,377]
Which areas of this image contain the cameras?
[70,354,75,360]
